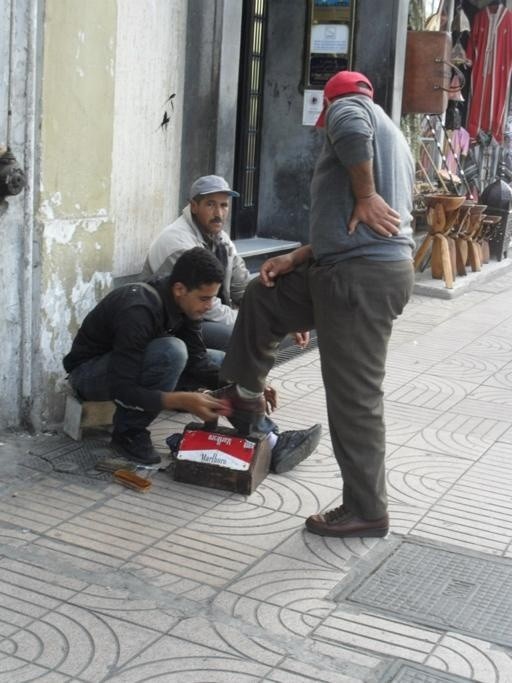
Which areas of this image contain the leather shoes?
[306,505,389,537]
[201,383,266,422]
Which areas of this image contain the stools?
[61,395,117,443]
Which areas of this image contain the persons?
[60,244,324,475]
[138,172,311,356]
[192,68,418,540]
[444,105,472,176]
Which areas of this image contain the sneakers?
[108,428,161,463]
[272,423,321,474]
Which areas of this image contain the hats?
[315,71,375,129]
[189,174,240,201]
